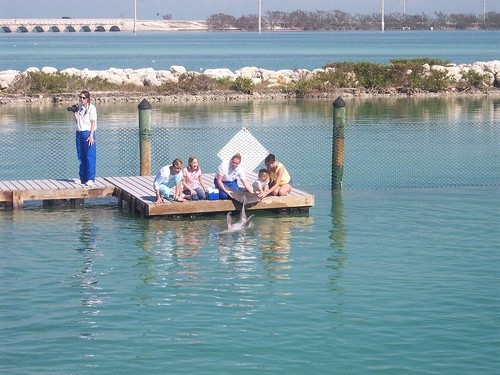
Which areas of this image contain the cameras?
[67,105,79,112]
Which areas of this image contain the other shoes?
[75,180,81,184]
[86,180,95,185]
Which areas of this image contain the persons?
[258,154,293,198]
[72,91,97,185]
[252,168,270,194]
[182,156,209,200]
[214,153,254,200]
[153,159,183,204]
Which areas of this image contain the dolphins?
[213,184,262,235]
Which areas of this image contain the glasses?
[80,96,86,99]
